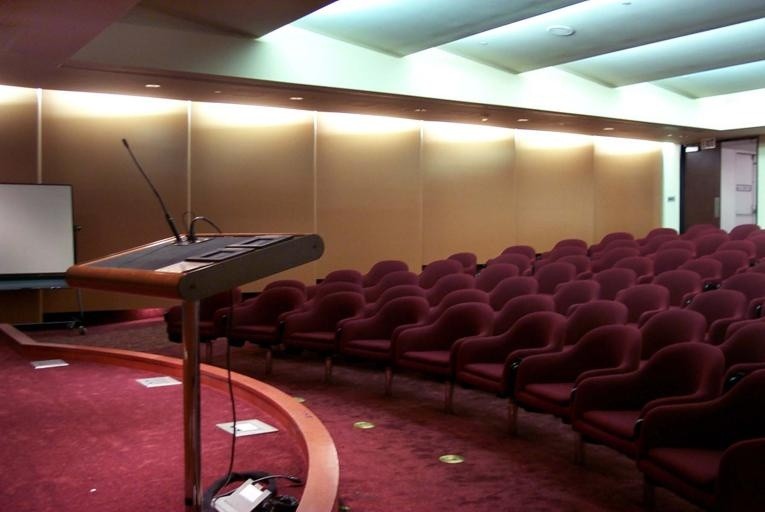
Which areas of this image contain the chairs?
[565,343,724,504]
[164,285,241,365]
[714,321,763,392]
[264,222,763,351]
[636,308,707,369]
[446,310,569,436]
[510,325,644,464]
[392,300,495,411]
[224,287,307,377]
[336,297,431,396]
[639,369,762,509]
[276,291,367,381]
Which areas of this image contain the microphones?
[121,138,182,242]
[210,475,302,512]
